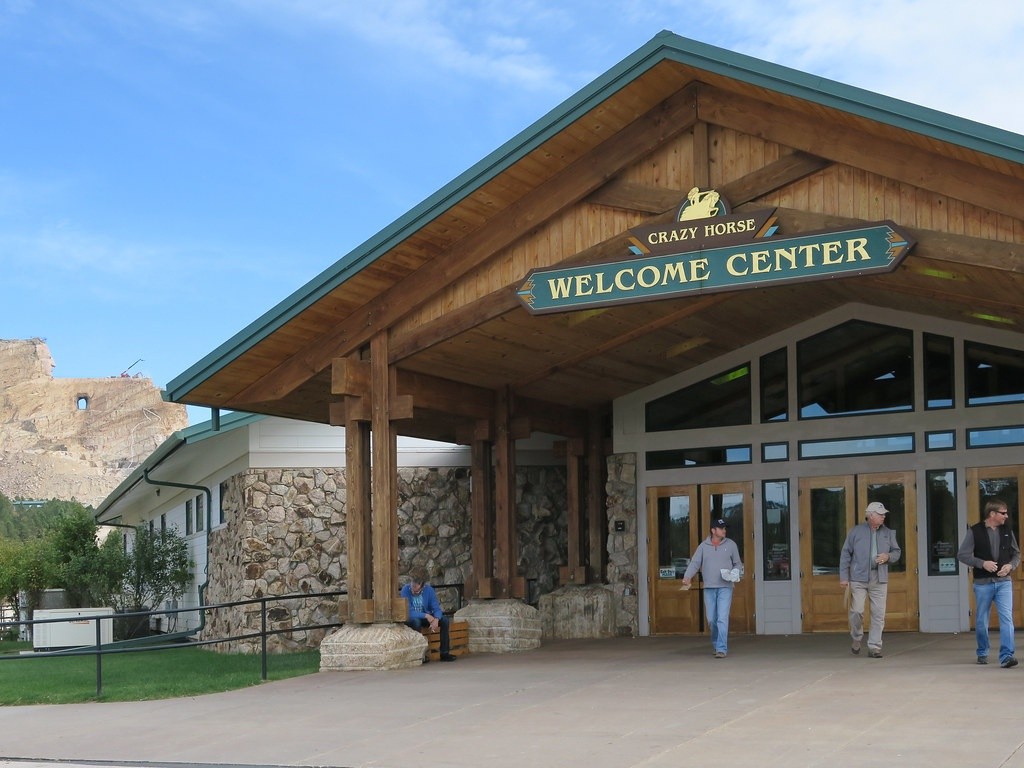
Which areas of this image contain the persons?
[840,502,901,658]
[401,578,457,663]
[957,499,1021,667]
[683,519,742,658]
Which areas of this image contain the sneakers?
[1001,657,1019,668]
[868,648,883,658]
[712,649,726,658]
[977,656,989,664]
[852,641,861,654]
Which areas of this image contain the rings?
[881,560,883,562]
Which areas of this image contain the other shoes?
[440,654,457,662]
[422,656,430,664]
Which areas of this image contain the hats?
[712,518,731,528]
[865,502,889,515]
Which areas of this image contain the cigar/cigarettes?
[432,630,435,633]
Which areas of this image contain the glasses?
[717,526,727,531]
[995,510,1008,515]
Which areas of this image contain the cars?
[669,558,691,577]
